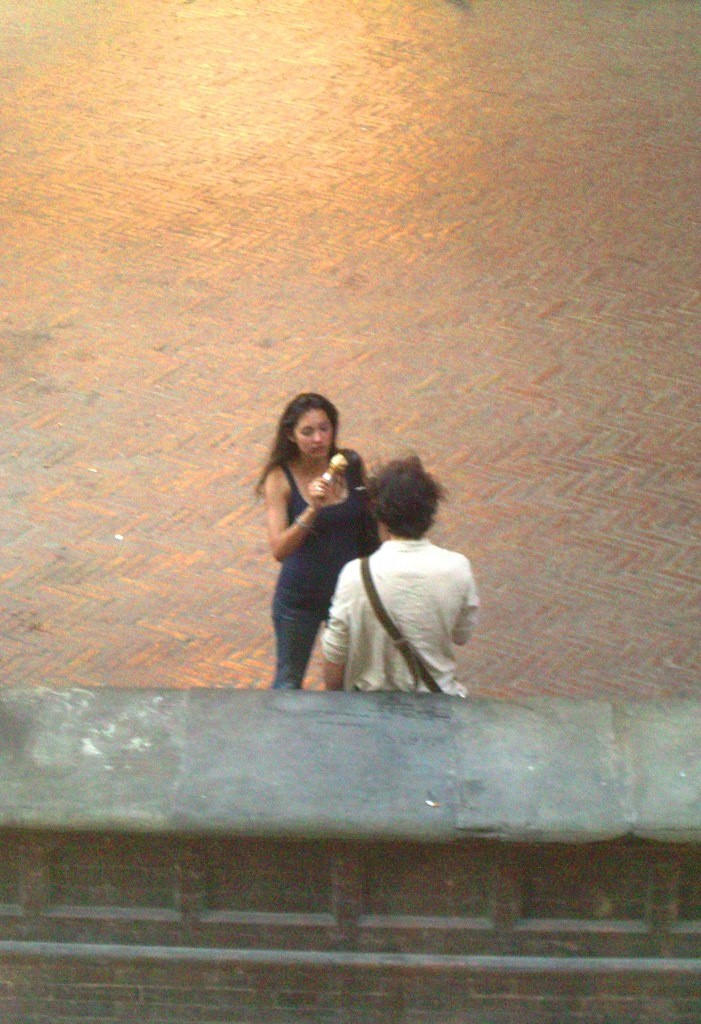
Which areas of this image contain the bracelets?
[296,517,309,529]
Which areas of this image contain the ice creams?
[324,453,348,478]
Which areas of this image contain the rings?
[315,486,321,491]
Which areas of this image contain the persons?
[255,395,378,689]
[322,456,477,698]
